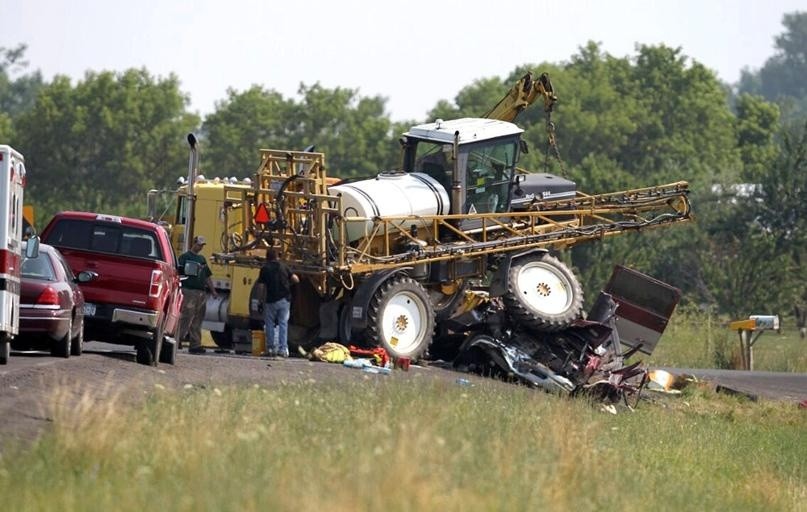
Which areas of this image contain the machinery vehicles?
[147,71,693,372]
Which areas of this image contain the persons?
[176,235,220,353]
[256,247,301,359]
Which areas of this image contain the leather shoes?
[278,349,289,357]
[260,352,278,357]
[189,346,206,353]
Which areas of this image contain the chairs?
[119,236,152,256]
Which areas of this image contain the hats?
[195,235,206,244]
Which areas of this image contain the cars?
[10,240,92,358]
[38,210,189,366]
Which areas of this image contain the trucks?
[0,144,40,363]
